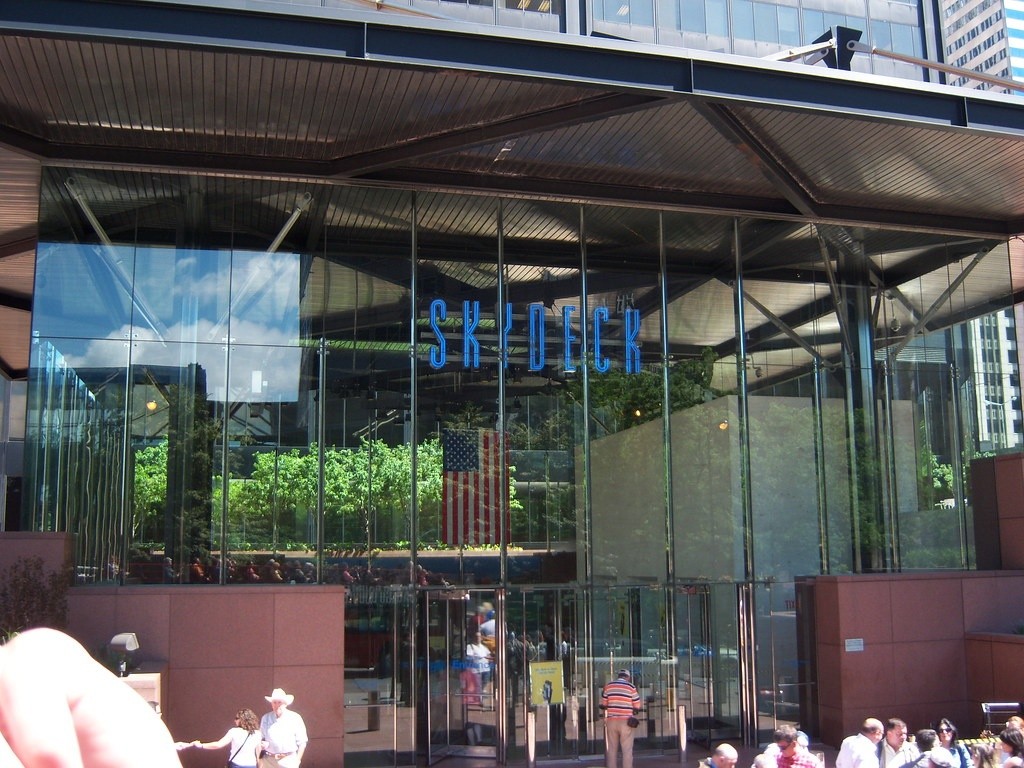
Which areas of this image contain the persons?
[1,628,1024,767]
[104,549,575,700]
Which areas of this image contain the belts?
[265,751,289,760]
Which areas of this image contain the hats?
[163,557,172,563]
[488,610,496,619]
[265,688,295,706]
[930,747,953,767]
[618,668,630,677]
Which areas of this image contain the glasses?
[939,727,952,733]
[780,745,790,750]
[235,717,240,720]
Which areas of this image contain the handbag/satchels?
[628,717,640,728]
[223,756,230,768]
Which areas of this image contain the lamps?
[749,354,764,378]
[146,400,158,410]
[890,301,901,331]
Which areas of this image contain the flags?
[442,427,511,546]
